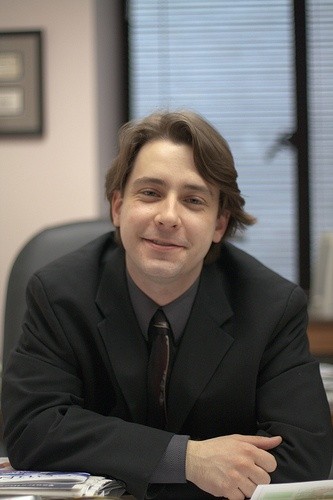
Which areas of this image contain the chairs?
[2,219,116,371]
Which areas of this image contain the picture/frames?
[0,28,43,138]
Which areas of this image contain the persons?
[2,110,333,500]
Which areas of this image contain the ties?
[147,320,169,431]
